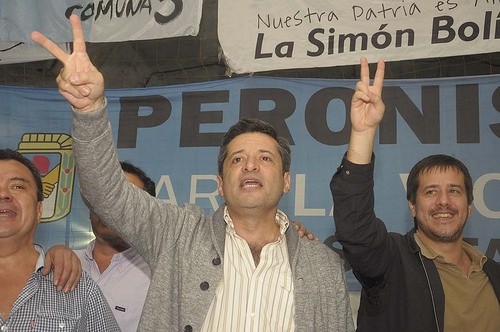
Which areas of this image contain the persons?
[73,158,320,332]
[31,14,356,332]
[42,244,82,294]
[329,56,500,332]
[0,148,122,332]
